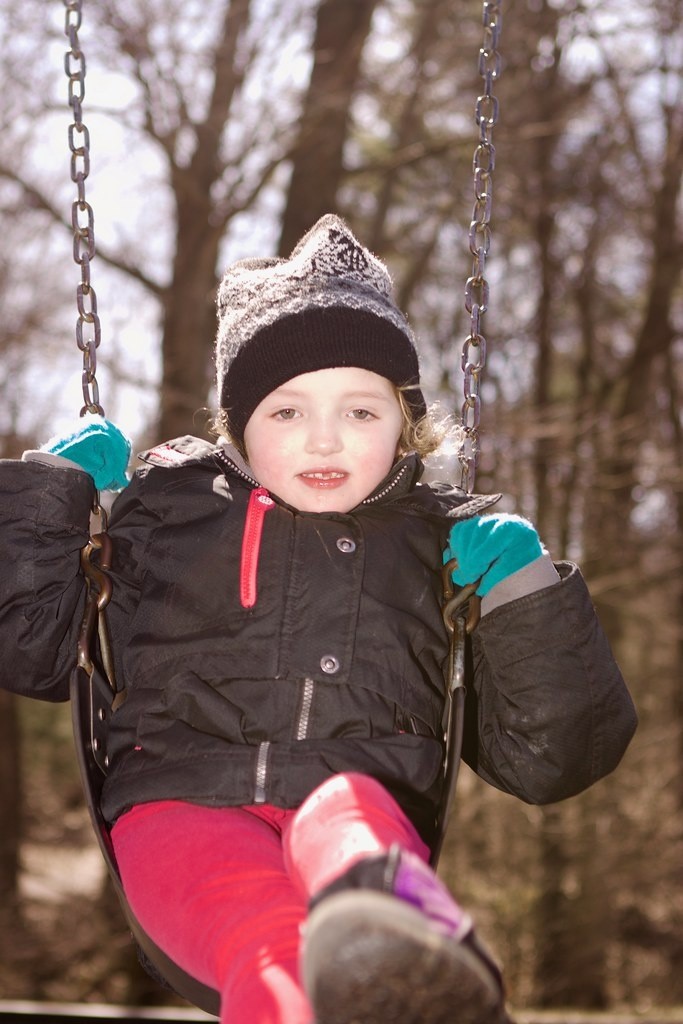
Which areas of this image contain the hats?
[217,215,427,465]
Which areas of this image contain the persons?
[0,213,641,1024]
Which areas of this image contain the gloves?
[443,513,547,596]
[37,415,132,492]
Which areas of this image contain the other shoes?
[297,891,508,1023]
